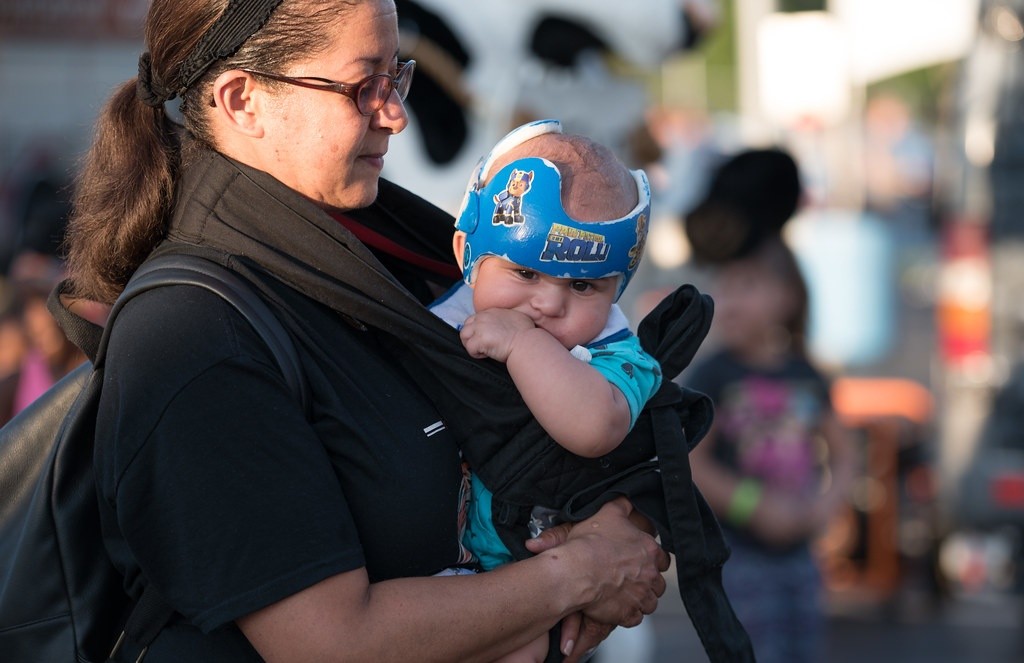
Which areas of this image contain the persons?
[428,117,648,663]
[1,1,669,663]
[618,0,1024,663]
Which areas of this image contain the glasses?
[227,58,417,116]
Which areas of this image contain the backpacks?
[0,254,303,663]
